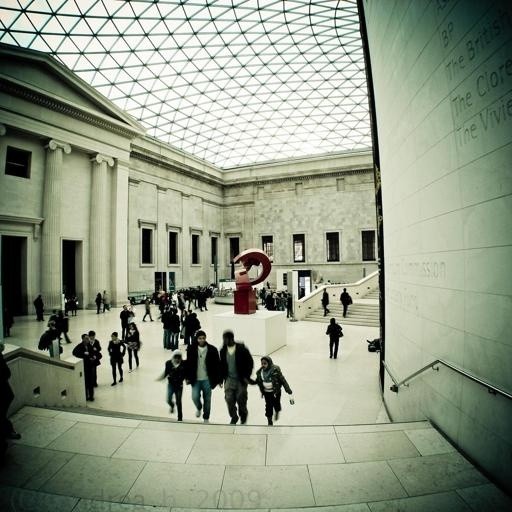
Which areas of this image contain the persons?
[33,281,353,426]
[4,306,15,337]
[0,345,21,454]
